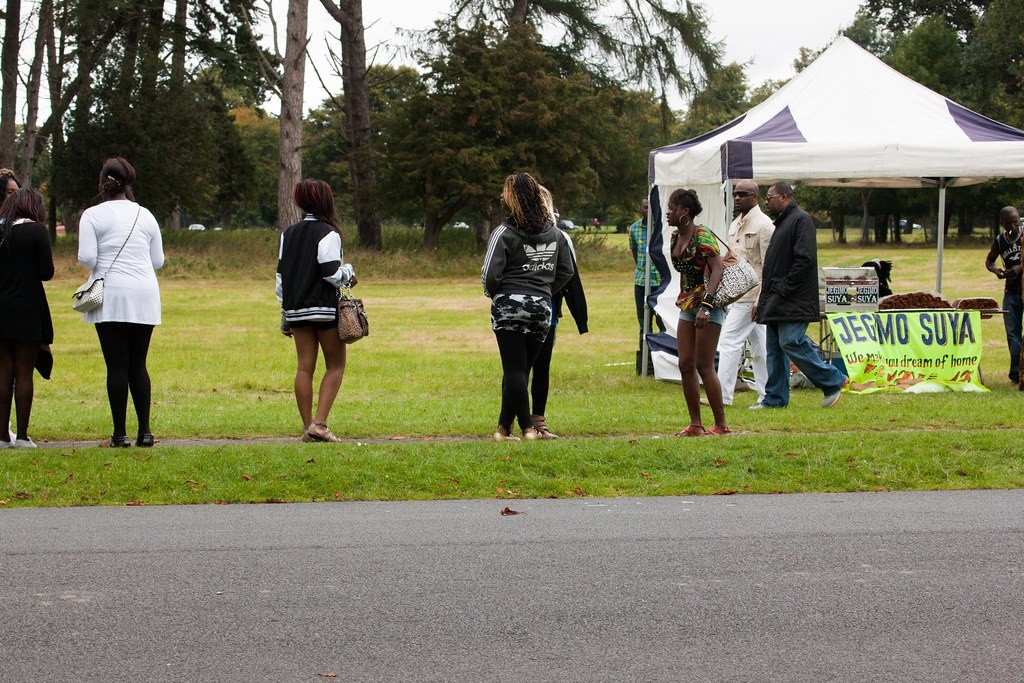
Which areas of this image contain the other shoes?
[524,429,543,440]
[531,414,558,439]
[493,432,521,442]
[99,436,131,448]
[748,404,763,410]
[135,433,154,447]
[822,376,849,409]
[699,398,710,406]
[1008,373,1019,384]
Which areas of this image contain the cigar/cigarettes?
[1009,230,1012,234]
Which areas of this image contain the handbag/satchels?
[695,226,759,310]
[72,276,104,312]
[337,283,369,345]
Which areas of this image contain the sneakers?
[15,436,37,448]
[0,438,15,448]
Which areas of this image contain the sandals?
[307,419,341,443]
[302,430,319,443]
[698,425,730,438]
[675,424,705,438]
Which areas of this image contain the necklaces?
[1006,232,1019,244]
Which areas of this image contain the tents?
[638,35,1024,379]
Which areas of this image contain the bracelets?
[699,292,716,316]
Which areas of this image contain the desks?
[816,308,1010,389]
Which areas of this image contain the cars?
[452,222,469,228]
[560,220,578,230]
[188,224,205,231]
[889,220,921,229]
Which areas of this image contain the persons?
[861,258,893,297]
[985,206,1024,390]
[629,198,661,374]
[696,179,776,408]
[592,218,600,225]
[0,168,55,450]
[666,189,732,438]
[276,179,358,442]
[78,159,164,447]
[748,183,847,412]
[481,173,589,442]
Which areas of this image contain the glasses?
[732,191,757,198]
[764,193,782,202]
